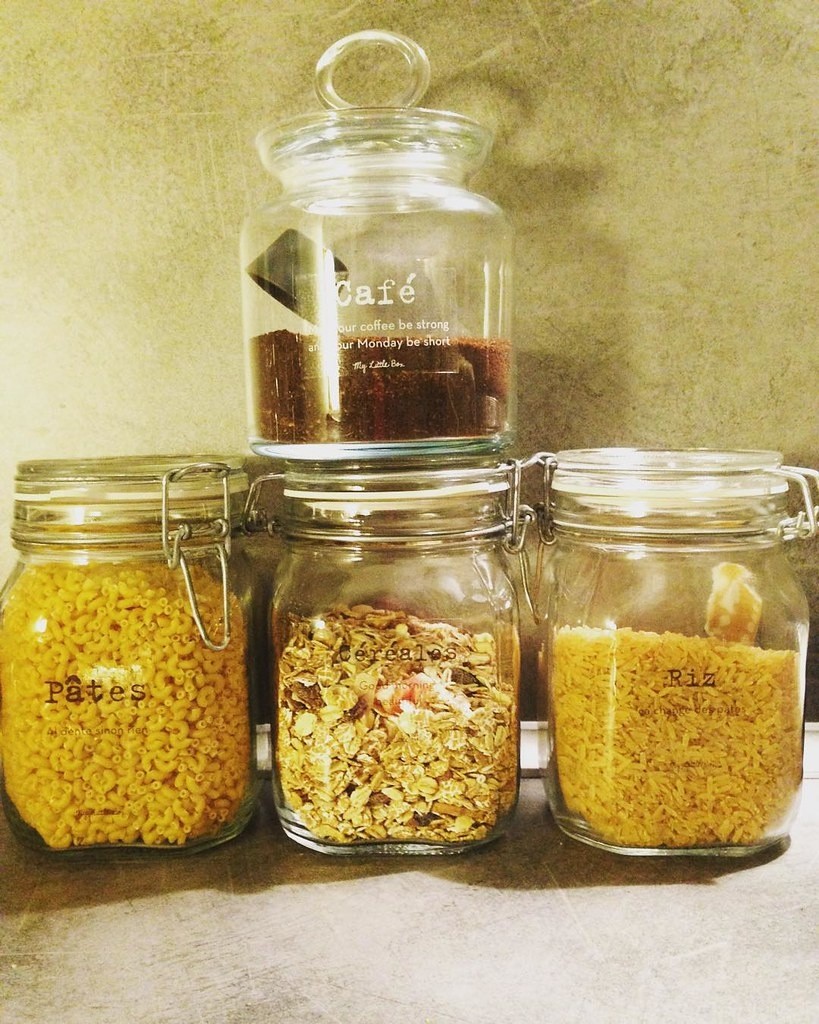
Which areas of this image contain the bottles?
[533,444,819,861]
[243,448,538,858]
[0,454,268,867]
[239,30,517,459]
